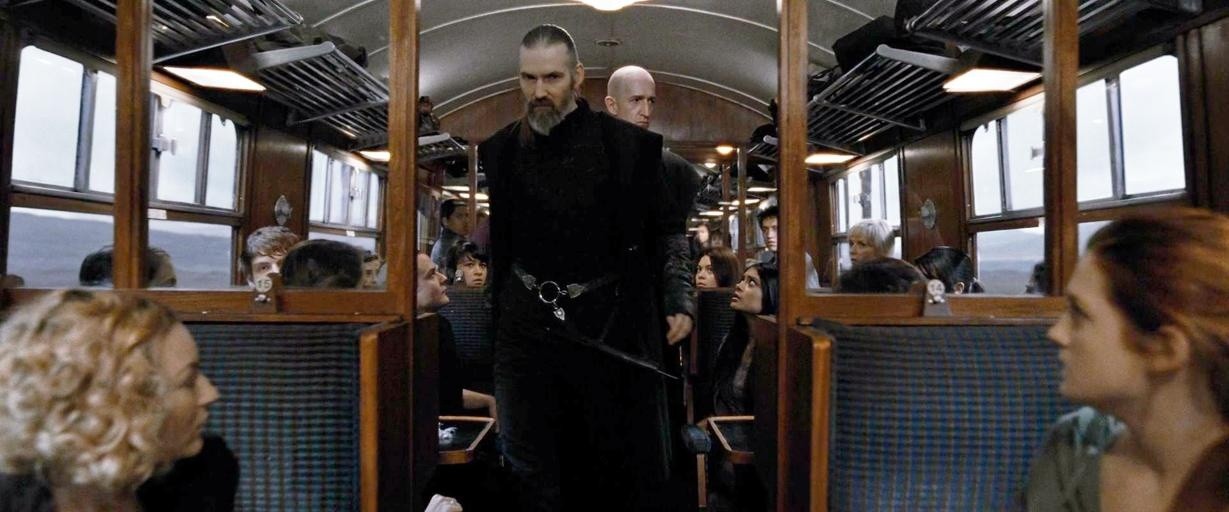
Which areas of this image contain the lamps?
[161,47,272,104]
[943,52,1045,101]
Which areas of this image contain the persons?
[1012,205,1228,512]
[0,285,240,512]
[81,248,177,288]
[604,64,703,427]
[247,228,383,289]
[1025,252,1058,303]
[842,221,984,295]
[414,201,501,435]
[478,22,664,511]
[688,208,820,451]
